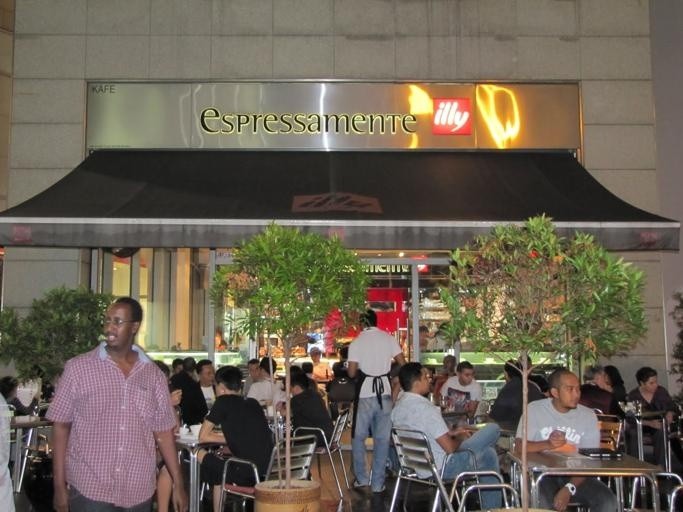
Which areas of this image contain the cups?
[180,425,200,436]
[621,400,642,415]
[429,392,451,410]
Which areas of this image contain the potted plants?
[428,210,651,511]
[210,221,374,511]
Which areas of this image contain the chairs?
[0,375,683,512]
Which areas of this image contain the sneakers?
[372,484,386,492]
[351,478,370,488]
